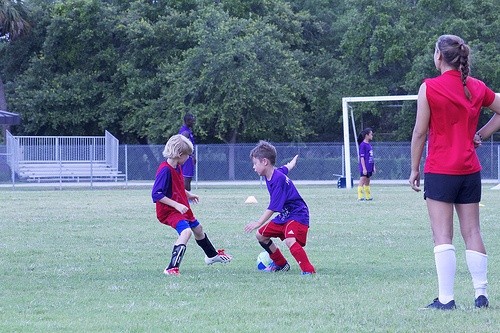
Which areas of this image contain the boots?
[357,186,364,200]
[364,185,372,200]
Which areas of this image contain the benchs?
[9,160,126,182]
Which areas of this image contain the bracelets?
[476,131,484,142]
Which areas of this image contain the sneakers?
[164,267,181,277]
[475,295,488,308]
[425,297,456,310]
[303,272,312,276]
[205,250,232,265]
[264,261,290,272]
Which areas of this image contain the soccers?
[257,252,273,271]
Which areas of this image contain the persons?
[177,114,197,202]
[151,134,232,278]
[408,34,500,311]
[357,128,376,200]
[242,141,317,278]
